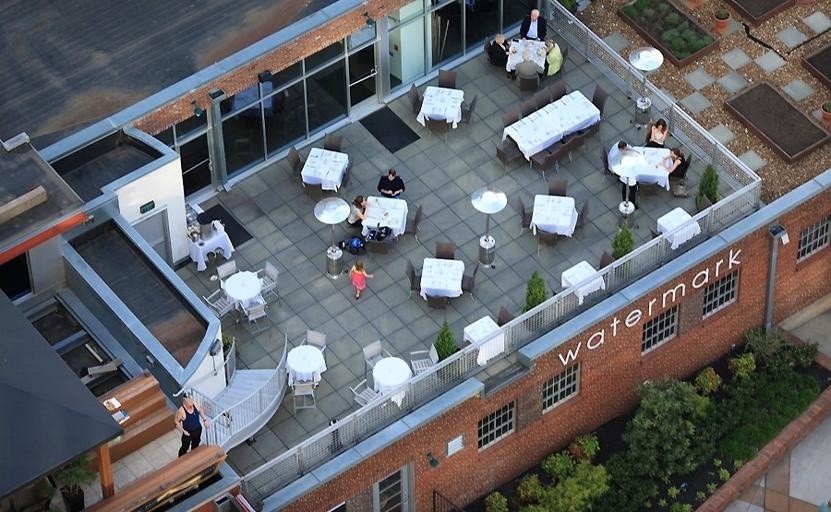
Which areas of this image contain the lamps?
[366,19,375,27]
[207,88,226,105]
[429,459,439,466]
[192,109,204,118]
[209,338,222,356]
[257,69,272,84]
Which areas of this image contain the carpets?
[198,204,253,248]
[358,106,422,151]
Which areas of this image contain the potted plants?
[611,220,635,280]
[694,164,724,224]
[521,271,550,333]
[433,321,459,386]
[821,100,831,122]
[53,451,98,510]
[714,8,732,29]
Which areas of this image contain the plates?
[511,49,517,54]
[527,42,534,47]
[382,211,389,219]
[537,47,546,57]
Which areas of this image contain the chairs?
[286,145,305,183]
[534,87,553,110]
[646,121,656,142]
[550,79,569,101]
[563,132,586,163]
[347,379,382,408]
[301,180,325,203]
[495,307,515,328]
[548,179,567,195]
[461,95,479,125]
[666,154,692,196]
[685,194,713,216]
[599,145,616,185]
[427,117,451,145]
[547,141,574,169]
[455,341,469,351]
[537,230,560,258]
[404,260,422,299]
[407,83,423,117]
[324,134,343,151]
[591,84,609,121]
[294,383,316,415]
[254,261,281,308]
[520,97,538,118]
[341,161,355,189]
[593,251,612,274]
[436,242,456,259]
[644,224,662,239]
[546,279,562,295]
[439,68,457,89]
[484,38,496,65]
[299,329,327,362]
[216,260,241,283]
[202,289,237,326]
[529,149,560,183]
[583,119,600,145]
[238,296,273,336]
[518,77,540,92]
[425,293,449,320]
[500,109,521,144]
[408,344,439,378]
[405,204,423,246]
[463,263,480,303]
[366,240,398,255]
[576,201,591,237]
[362,339,393,378]
[550,45,569,79]
[491,139,525,174]
[512,195,535,236]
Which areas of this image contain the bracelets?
[203,419,207,422]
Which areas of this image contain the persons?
[489,34,516,67]
[377,169,405,200]
[347,195,370,228]
[644,118,668,148]
[658,147,687,177]
[174,391,209,458]
[619,176,639,209]
[608,140,640,175]
[536,40,563,76]
[349,260,373,301]
[520,9,547,42]
[515,49,543,88]
